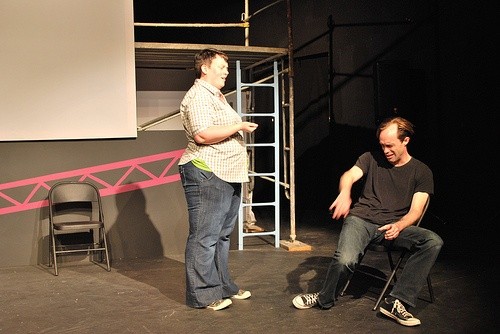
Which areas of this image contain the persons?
[178,48,258,311]
[292,117,443,326]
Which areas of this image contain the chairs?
[340,196,435,311]
[48,181,111,276]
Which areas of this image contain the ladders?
[236,60,280,250]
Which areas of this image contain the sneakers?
[379,297,421,327]
[207,299,233,311]
[228,287,253,301]
[291,292,328,309]
